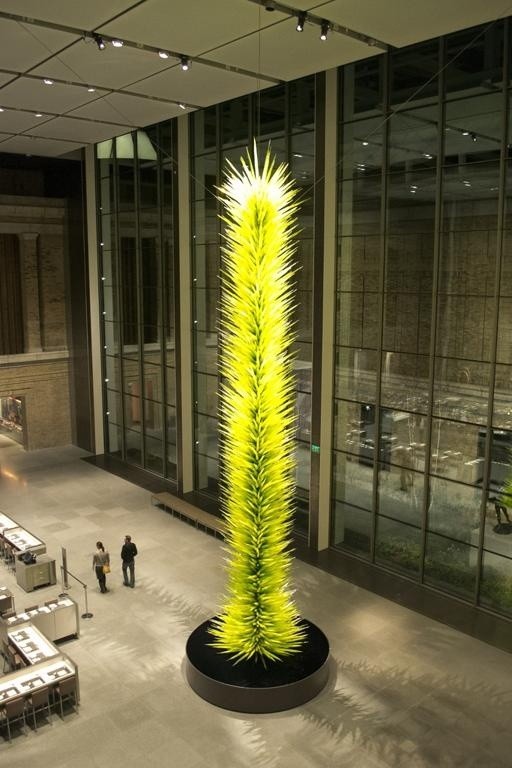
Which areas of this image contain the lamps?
[179,53,188,71]
[294,8,331,43]
[93,31,107,53]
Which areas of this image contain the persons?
[92,541,111,593]
[120,536,137,589]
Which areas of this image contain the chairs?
[0,508,82,742]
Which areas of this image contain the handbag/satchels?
[102,564,110,573]
